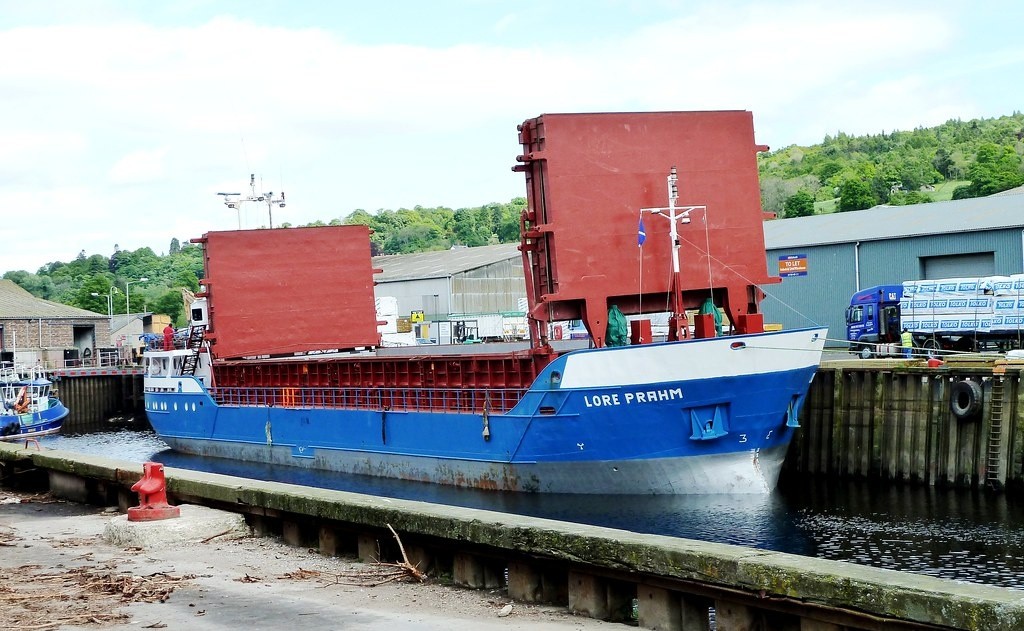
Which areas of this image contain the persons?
[928,356,944,367]
[163,323,175,351]
[900,328,914,359]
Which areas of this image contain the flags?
[637,220,646,247]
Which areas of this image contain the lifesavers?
[950,379,983,419]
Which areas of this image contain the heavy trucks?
[844,273,1024,361]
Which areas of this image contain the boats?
[138,109,830,497]
[0,365,70,442]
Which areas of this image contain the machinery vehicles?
[450,320,483,345]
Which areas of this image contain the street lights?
[91,286,119,330]
[127,278,149,352]
